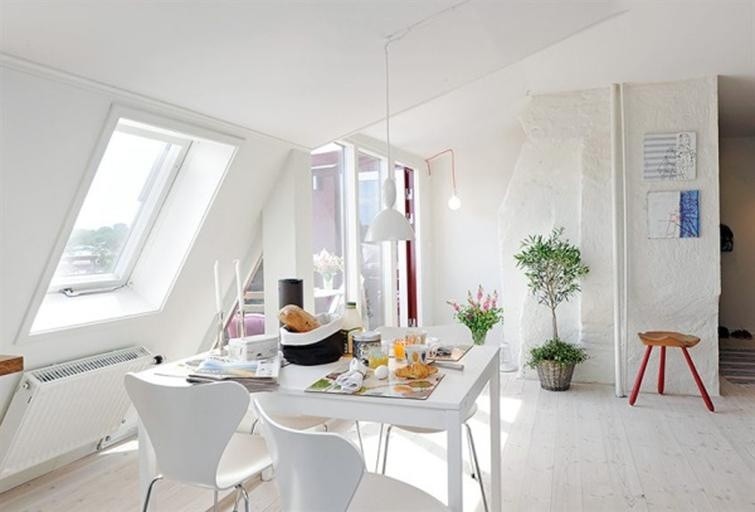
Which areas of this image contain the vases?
[323,274,333,295]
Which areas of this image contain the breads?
[281,302,319,335]
[399,362,438,379]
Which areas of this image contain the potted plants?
[512,225,591,390]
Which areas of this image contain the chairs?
[252,398,450,511]
[250,414,364,472]
[124,373,327,512]
[373,324,490,511]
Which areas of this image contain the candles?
[235,260,244,312]
[214,260,224,315]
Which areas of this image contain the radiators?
[0,343,155,479]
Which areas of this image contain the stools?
[629,331,715,411]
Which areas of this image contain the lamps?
[426,148,460,211]
[362,30,417,242]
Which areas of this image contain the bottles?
[339,302,363,358]
[352,333,382,362]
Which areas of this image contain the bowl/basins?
[425,337,440,364]
[279,311,343,366]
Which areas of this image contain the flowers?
[314,248,343,282]
[446,284,505,345]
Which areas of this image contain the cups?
[392,335,412,360]
[404,327,427,344]
[277,278,303,351]
[369,346,391,369]
[404,342,427,366]
